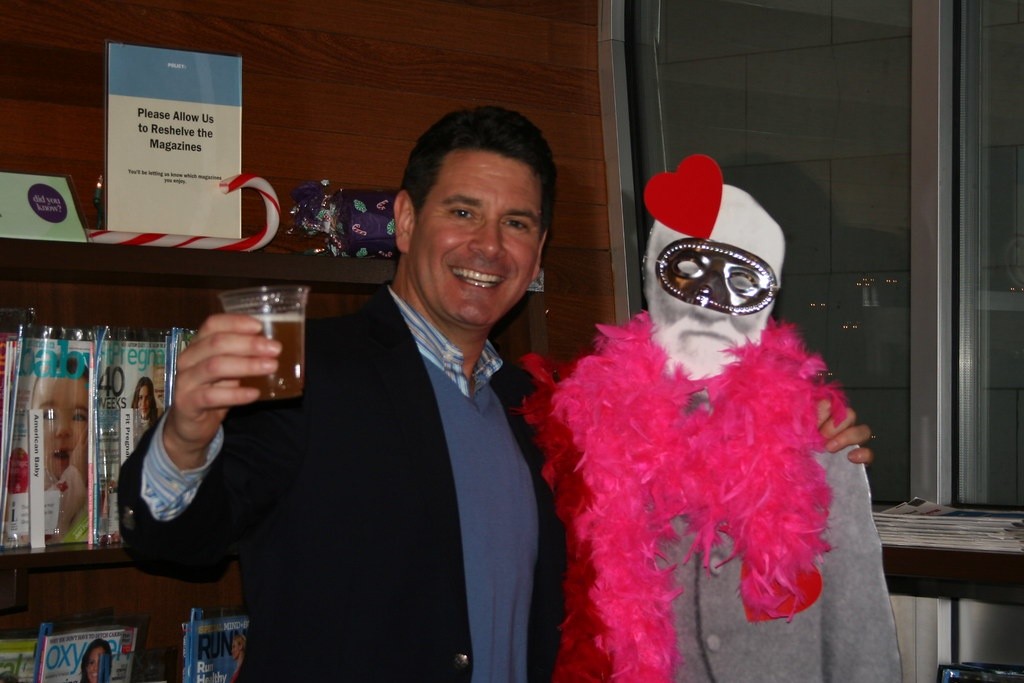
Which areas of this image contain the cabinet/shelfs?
[1,238,552,683]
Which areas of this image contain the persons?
[117,107,873,683]
[534,153,900,682]
[77,640,112,683]
[30,359,88,541]
[132,377,157,428]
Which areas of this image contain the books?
[0,335,196,545]
[873,498,1024,553]
[38,625,137,683]
[181,614,249,683]
[0,629,39,683]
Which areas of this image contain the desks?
[882,545,1024,588]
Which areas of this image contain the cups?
[217,286,308,400]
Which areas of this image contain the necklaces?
[511,319,846,682]
